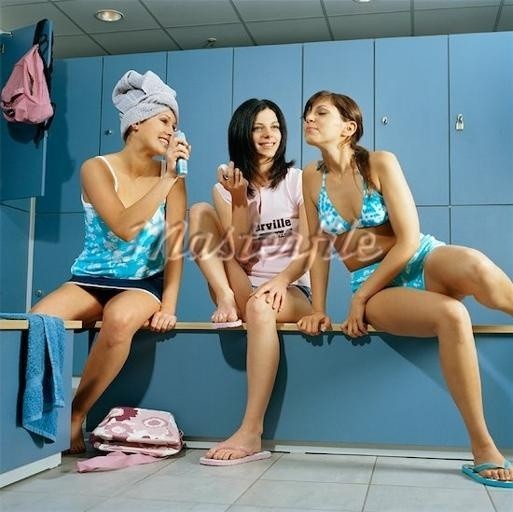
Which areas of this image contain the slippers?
[459,454,513,490]
[210,301,243,333]
[197,441,271,467]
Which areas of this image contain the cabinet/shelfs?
[0,19,513,377]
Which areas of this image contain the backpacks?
[0,34,55,126]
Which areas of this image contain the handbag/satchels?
[89,403,184,457]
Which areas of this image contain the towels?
[0,313,66,443]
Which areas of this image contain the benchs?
[0,317,513,486]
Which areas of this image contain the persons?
[295,88,512,485]
[27,69,188,456]
[188,97,312,466]
